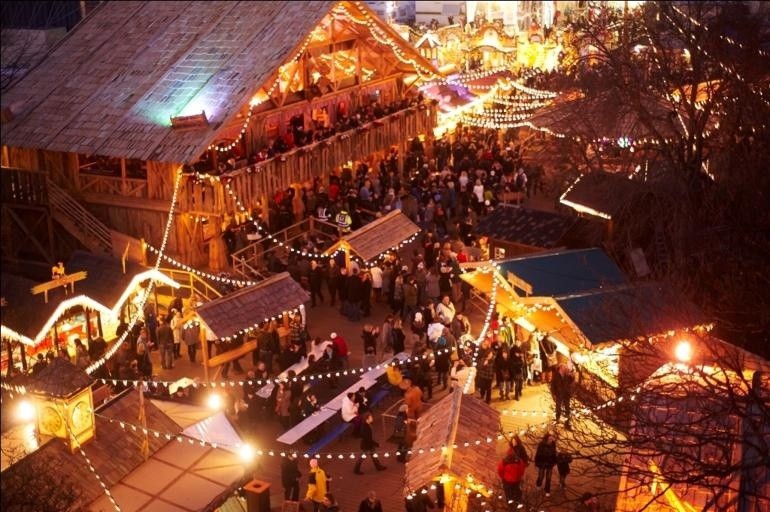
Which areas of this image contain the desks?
[276,340,411,447]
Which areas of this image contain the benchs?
[301,422,353,465]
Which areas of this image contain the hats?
[398,403,408,413]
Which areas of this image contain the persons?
[0,6,610,511]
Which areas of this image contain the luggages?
[282,498,301,512]
